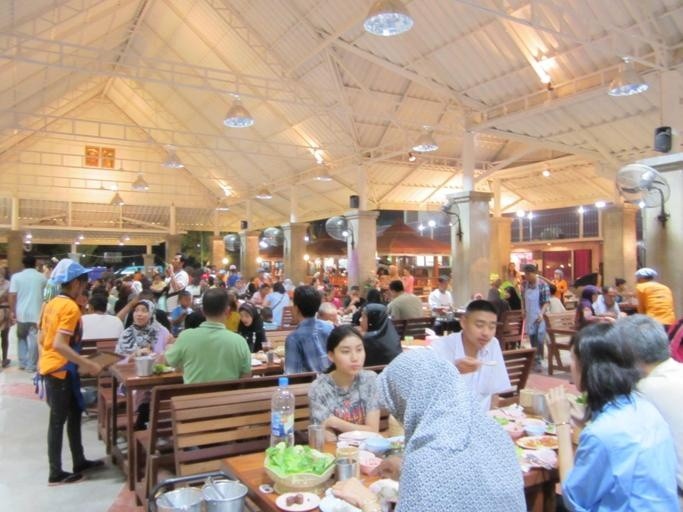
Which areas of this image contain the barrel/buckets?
[201,478,249,512]
[520,389,553,424]
[133,352,160,376]
[155,486,204,512]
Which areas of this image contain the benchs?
[37,286,641,511]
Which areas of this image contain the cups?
[135,355,156,376]
[307,422,404,478]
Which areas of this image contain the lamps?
[214,197,231,213]
[221,92,256,130]
[157,147,186,170]
[604,55,650,99]
[308,162,333,181]
[409,125,441,155]
[254,183,273,201]
[129,174,150,190]
[361,1,415,37]
[108,190,125,207]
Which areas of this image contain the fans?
[611,162,672,226]
[424,193,465,243]
[222,233,245,265]
[322,213,356,249]
[259,226,289,256]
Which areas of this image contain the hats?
[634,267,658,278]
[48,259,93,285]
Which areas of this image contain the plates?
[484,387,589,472]
[152,367,174,373]
[274,478,399,512]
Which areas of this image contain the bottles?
[269,377,293,448]
[403,329,453,346]
[250,331,284,367]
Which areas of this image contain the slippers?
[48,461,105,485]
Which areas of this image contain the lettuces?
[265,444,337,474]
[154,365,167,375]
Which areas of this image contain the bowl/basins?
[263,446,335,497]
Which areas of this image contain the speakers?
[350,195,359,208]
[655,126,671,152]
[242,220,247,229]
